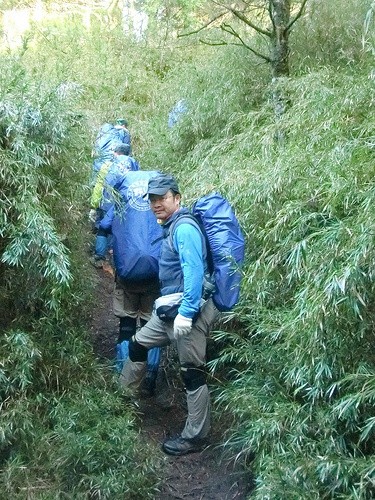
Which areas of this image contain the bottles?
[201,281,215,301]
[109,249,116,270]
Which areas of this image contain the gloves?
[172,313,192,340]
[89,209,98,222]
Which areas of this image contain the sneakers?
[164,436,203,455]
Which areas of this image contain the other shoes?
[141,378,155,395]
[90,257,103,268]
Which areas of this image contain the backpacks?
[94,122,123,157]
[168,192,245,312]
[100,158,139,211]
[111,171,165,279]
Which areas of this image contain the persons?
[88,142,140,260]
[115,175,215,455]
[89,119,131,188]
[97,204,160,395]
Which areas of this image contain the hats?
[116,118,127,126]
[115,144,130,155]
[143,174,180,196]
